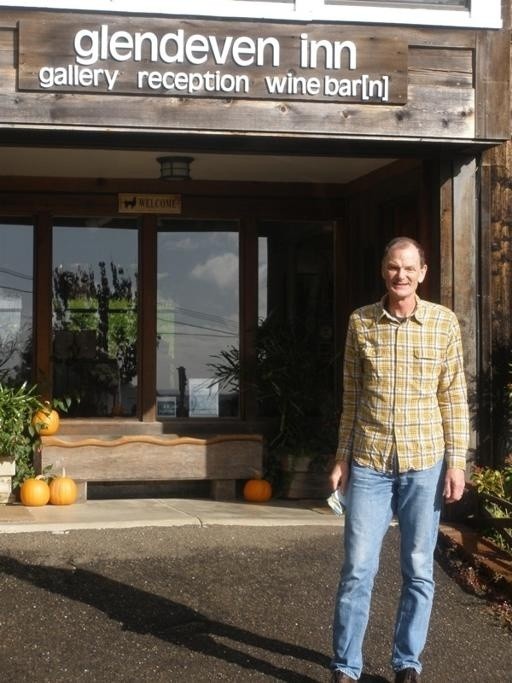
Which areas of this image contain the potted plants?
[0,380,82,504]
[205,301,346,501]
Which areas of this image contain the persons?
[328,236,470,683]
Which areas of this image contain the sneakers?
[394,665,422,683]
[329,667,358,683]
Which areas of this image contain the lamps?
[155,156,195,183]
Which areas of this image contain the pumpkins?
[32,408,60,435]
[49,477,78,505]
[243,479,273,502]
[20,479,50,505]
